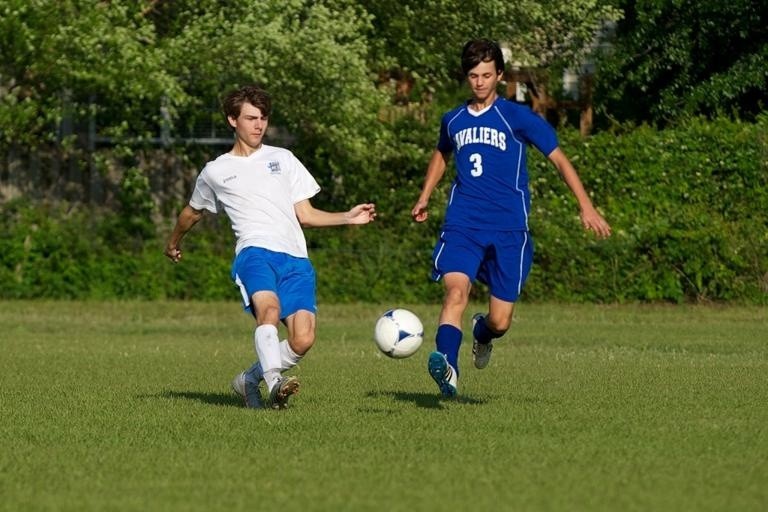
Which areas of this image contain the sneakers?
[268,373,300,411]
[471,312,496,370]
[424,349,460,400]
[230,368,267,411]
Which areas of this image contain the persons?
[163,85,379,412]
[408,38,613,401]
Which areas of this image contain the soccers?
[374,308,423,358]
